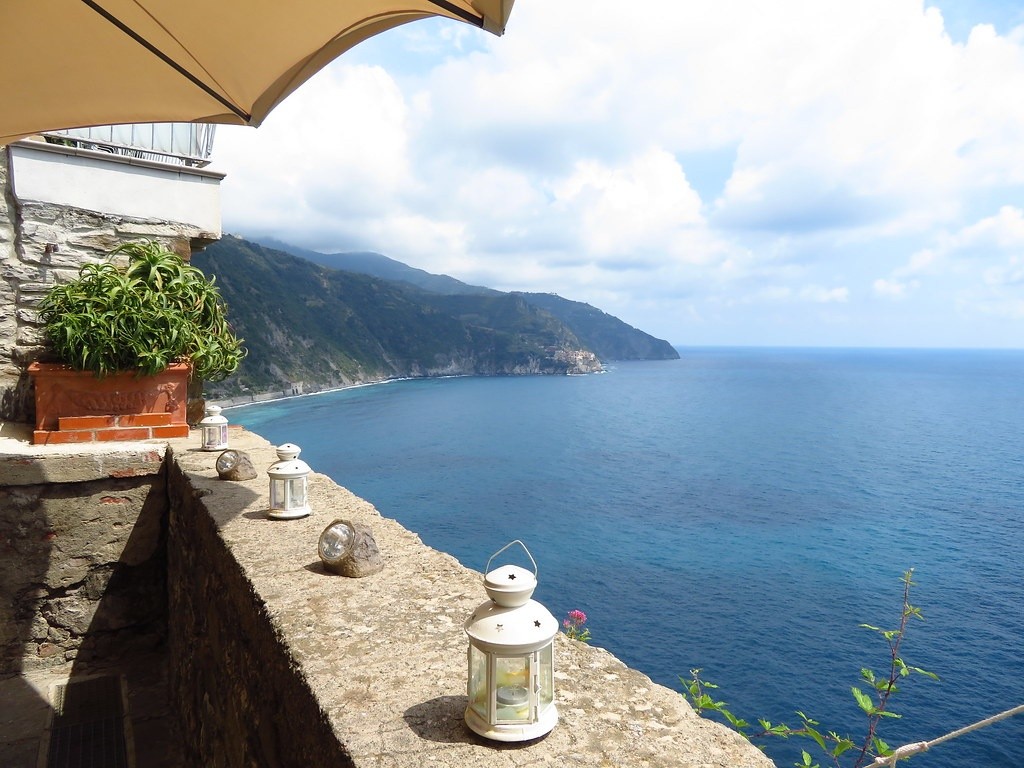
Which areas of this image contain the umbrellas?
[0,0,514,152]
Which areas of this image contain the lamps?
[264,442,312,520]
[463,539,558,743]
[199,405,229,452]
[215,449,258,482]
[317,519,384,579]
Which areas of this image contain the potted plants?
[25,237,251,432]
